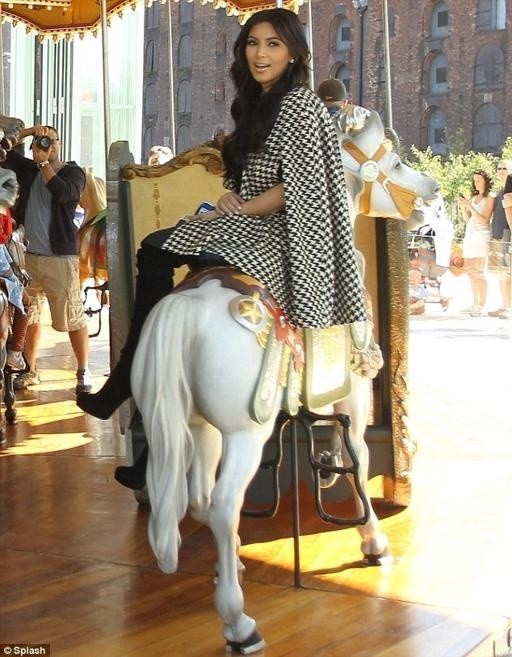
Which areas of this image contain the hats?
[316,79,352,102]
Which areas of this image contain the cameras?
[29,135,51,152]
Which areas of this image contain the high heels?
[77,361,141,430]
[115,445,147,490]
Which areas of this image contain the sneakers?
[459,304,510,318]
[6,350,27,371]
[76,370,93,393]
[14,371,41,389]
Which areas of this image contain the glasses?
[498,168,507,170]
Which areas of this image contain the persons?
[1,76,511,383]
[76,8,366,488]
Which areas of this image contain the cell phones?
[459,192,464,198]
